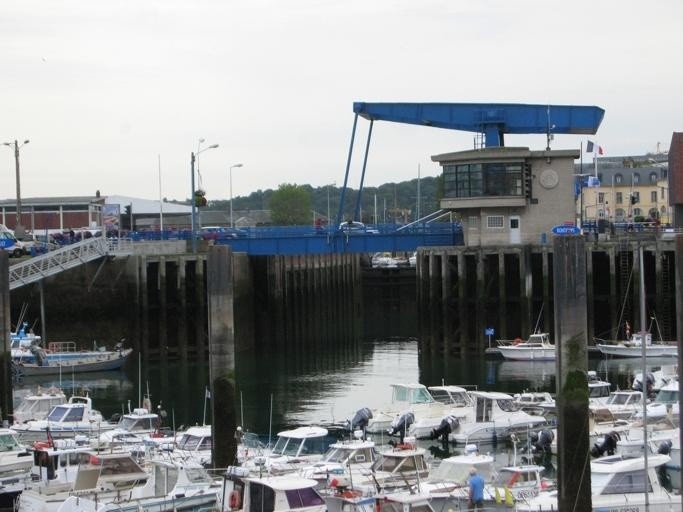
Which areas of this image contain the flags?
[585,141,603,155]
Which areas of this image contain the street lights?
[230,164,244,227]
[3,140,31,239]
[191,144,220,254]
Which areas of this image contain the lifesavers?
[228,490,241,510]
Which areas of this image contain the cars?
[197,227,249,239]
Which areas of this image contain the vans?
[0,224,24,258]
[20,234,60,254]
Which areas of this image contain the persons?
[68,227,74,245]
[315,218,322,229]
[467,467,485,511]
[626,215,634,231]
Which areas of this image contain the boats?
[11,332,133,376]
[597,330,678,357]
[372,252,416,270]
[495,332,556,360]
[1,364,683,512]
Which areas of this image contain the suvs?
[339,221,380,234]
